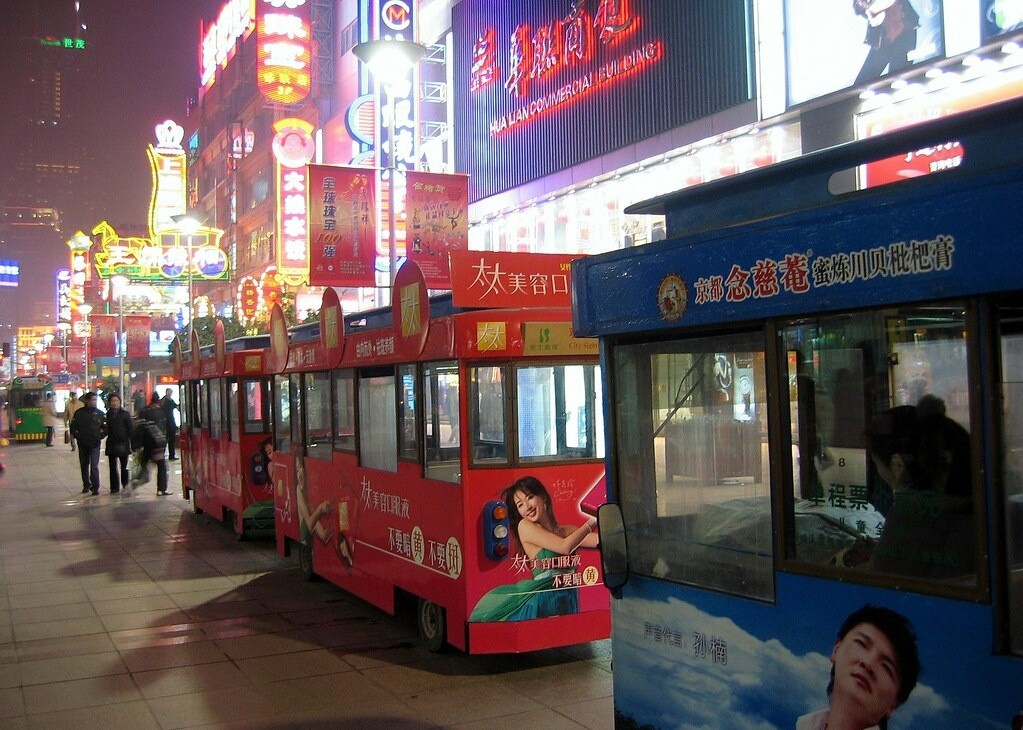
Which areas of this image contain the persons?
[500,477,600,620]
[42,393,57,447]
[796,602,919,730]
[63,389,179,496]
[294,452,333,545]
[854,0,919,84]
[863,405,979,579]
[259,436,274,483]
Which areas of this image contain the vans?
[172,320,270,543]
[6,374,54,440]
[267,251,612,668]
[570,98,1023,730]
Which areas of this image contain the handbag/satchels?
[65,430,70,444]
[126,448,151,490]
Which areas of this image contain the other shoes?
[157,490,173,496]
[47,445,54,446]
[92,488,100,495]
[111,490,117,493]
[169,457,179,461]
[83,487,89,493]
[72,448,76,451]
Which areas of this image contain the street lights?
[109,274,132,407]
[171,212,208,350]
[77,302,95,393]
[44,333,56,347]
[353,39,426,301]
[57,320,71,375]
